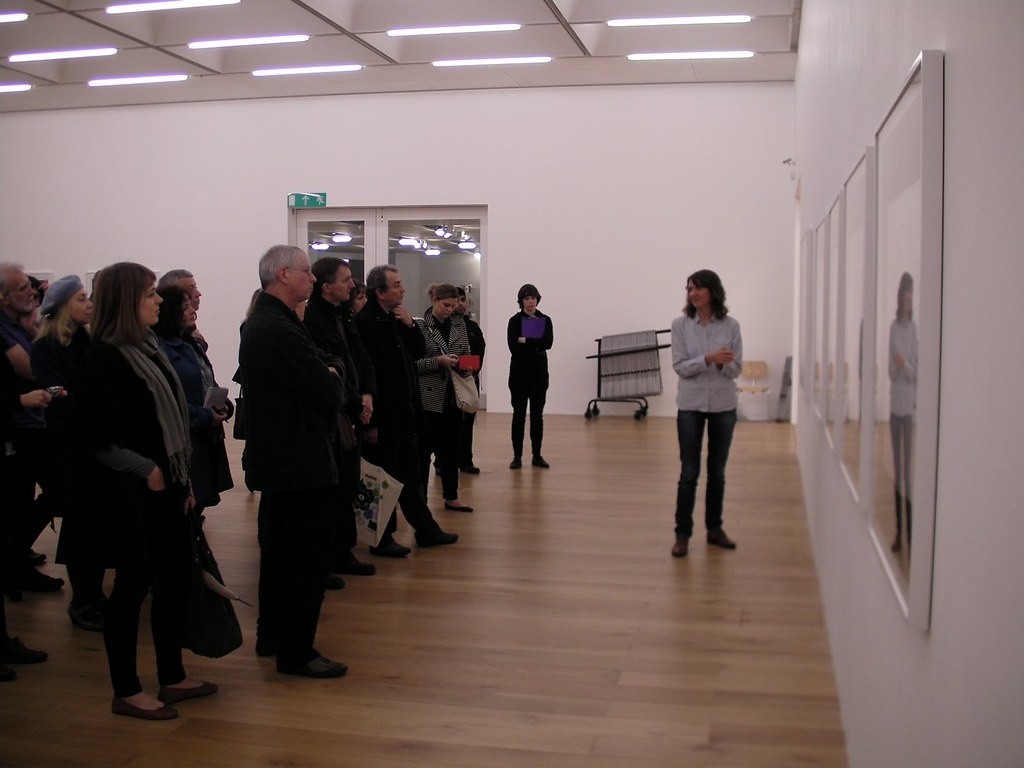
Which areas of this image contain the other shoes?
[445,502,473,512]
[891,534,902,550]
[707,529,735,548]
[532,455,549,467]
[436,468,441,475]
[417,531,458,547]
[322,575,344,590]
[370,540,410,557]
[331,555,375,575]
[461,463,479,473]
[510,456,521,469]
[0,552,108,682]
[672,534,687,555]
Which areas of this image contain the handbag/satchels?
[449,369,478,414]
[338,410,357,451]
[173,504,242,659]
[352,457,405,548]
[234,396,246,440]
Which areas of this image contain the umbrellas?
[203,568,255,609]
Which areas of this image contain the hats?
[40,275,83,317]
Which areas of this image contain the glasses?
[276,267,311,276]
[21,281,31,294]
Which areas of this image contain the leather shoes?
[256,638,279,655]
[112,695,177,720]
[158,682,217,704]
[278,655,347,677]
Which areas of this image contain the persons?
[507,284,553,470]
[672,270,742,558]
[411,284,486,513]
[887,273,919,552]
[0,263,234,720]
[232,245,459,678]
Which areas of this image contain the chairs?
[736,360,773,422]
